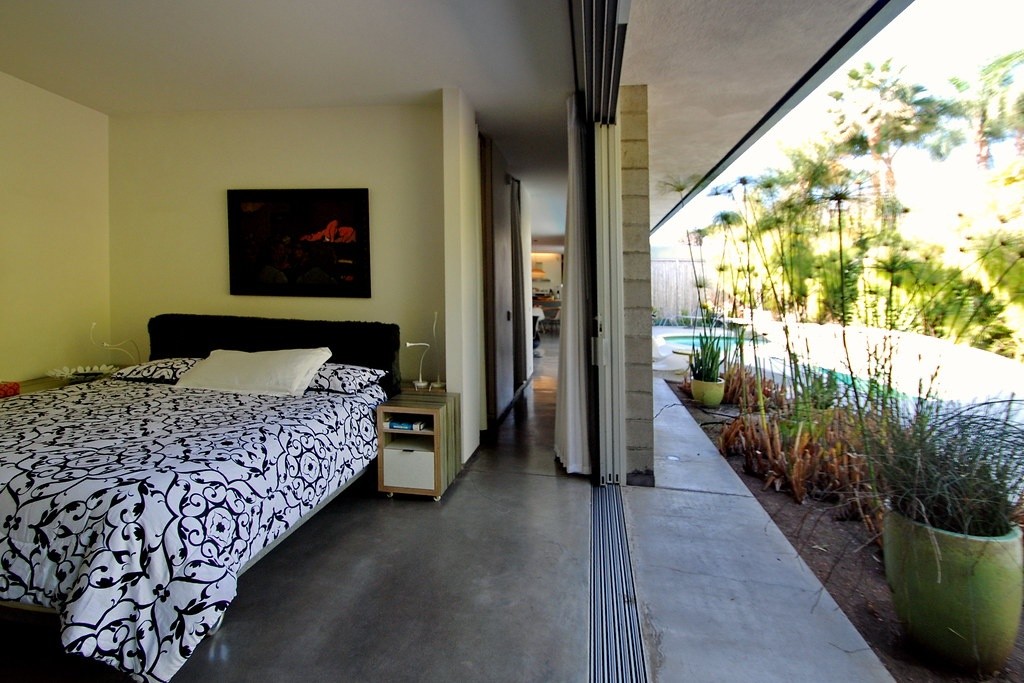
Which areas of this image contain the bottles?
[549,289,552,296]
[556,290,560,299]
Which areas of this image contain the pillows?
[175,347,332,396]
[307,362,388,394]
[111,357,203,385]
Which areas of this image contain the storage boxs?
[376,388,463,500]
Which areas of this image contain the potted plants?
[688,332,726,409]
[768,399,1023,668]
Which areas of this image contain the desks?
[532,298,561,321]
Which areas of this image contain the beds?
[0,314,401,683]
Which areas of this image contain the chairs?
[541,306,561,336]
